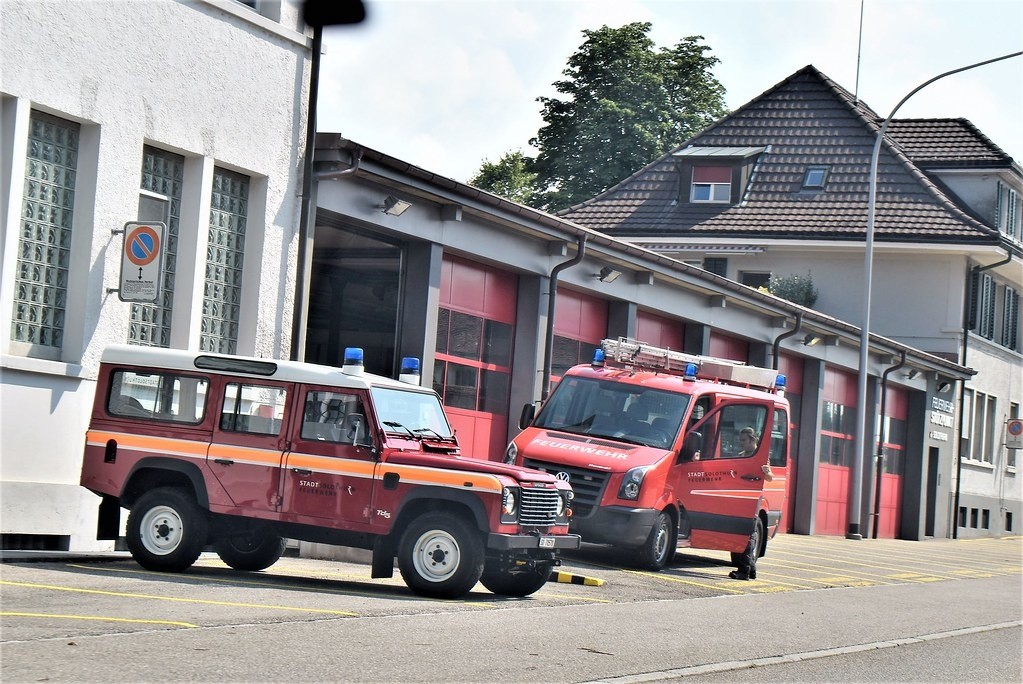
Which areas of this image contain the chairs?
[621,403,651,439]
[582,393,616,431]
[647,417,674,444]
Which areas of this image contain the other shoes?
[729,570,756,581]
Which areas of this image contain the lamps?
[937,381,950,393]
[903,369,923,379]
[376,195,411,216]
[592,266,621,283]
[798,334,821,346]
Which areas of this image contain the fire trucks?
[504,333,792,571]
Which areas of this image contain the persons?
[728,427,773,581]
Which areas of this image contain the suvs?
[79,342,582,600]
